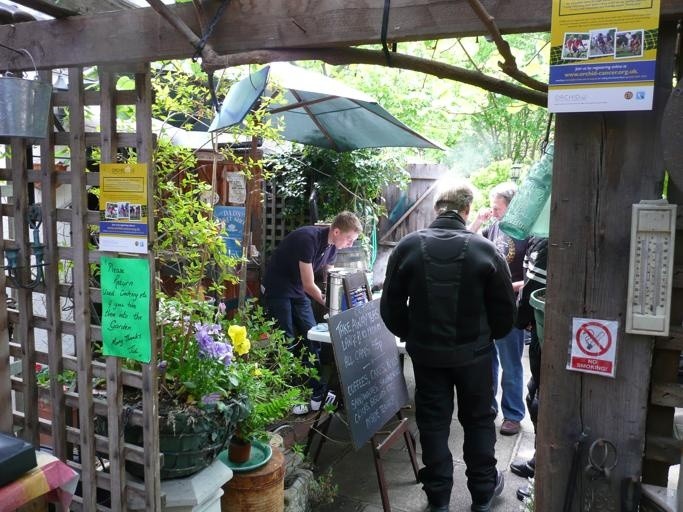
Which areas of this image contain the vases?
[113,407,236,487]
[529,286,546,350]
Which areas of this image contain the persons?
[265,211,364,415]
[509,236,548,502]
[565,31,640,57]
[379,182,518,512]
[466,182,529,435]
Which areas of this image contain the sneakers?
[507,456,535,501]
[472,469,505,511]
[498,418,523,436]
[290,389,343,415]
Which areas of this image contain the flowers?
[152,289,251,407]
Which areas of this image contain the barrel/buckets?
[325,269,373,323]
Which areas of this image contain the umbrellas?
[208,61,446,281]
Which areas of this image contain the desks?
[307,303,420,375]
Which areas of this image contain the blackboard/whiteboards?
[328,298,409,453]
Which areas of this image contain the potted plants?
[230,305,320,464]
